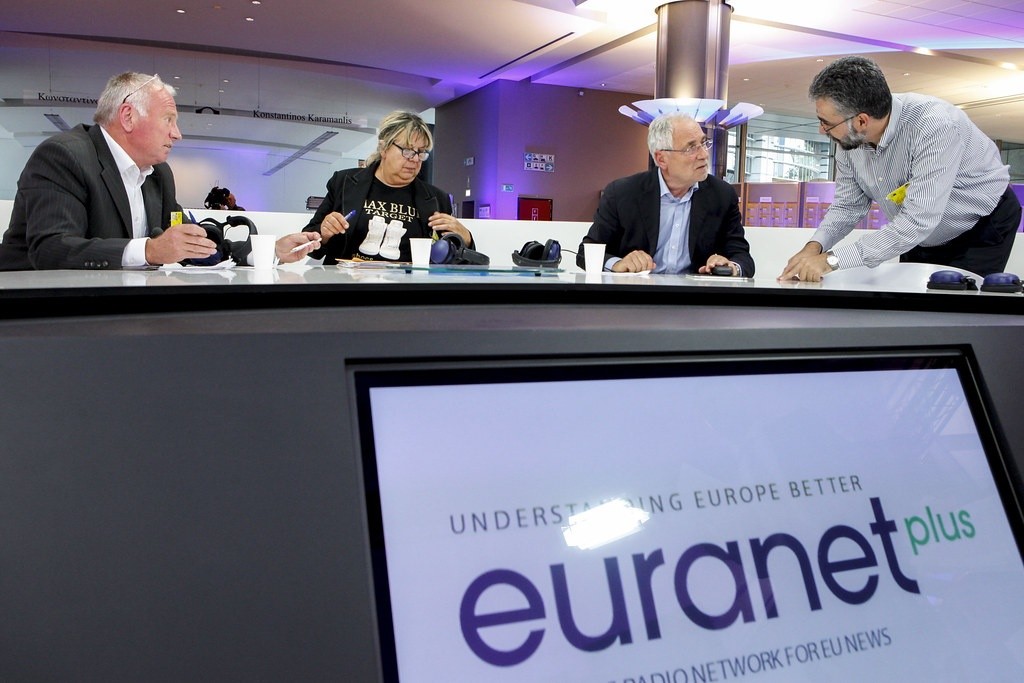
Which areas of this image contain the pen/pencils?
[289,238,323,253]
[386,264,432,270]
[188,210,221,260]
[345,210,356,221]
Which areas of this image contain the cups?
[582,242,608,278]
[249,234,277,272]
[409,237,434,269]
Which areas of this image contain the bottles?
[726,169,735,184]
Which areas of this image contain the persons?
[302,112,476,265]
[576,114,755,278]
[0,72,321,270]
[220,194,245,211]
[776,55,1022,281]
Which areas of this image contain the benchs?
[1,198,1024,275]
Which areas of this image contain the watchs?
[827,250,839,270]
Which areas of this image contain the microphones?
[151,227,187,267]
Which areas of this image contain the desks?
[0,264,1024,683]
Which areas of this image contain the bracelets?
[733,264,740,274]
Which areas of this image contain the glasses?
[820,111,869,135]
[394,143,430,161]
[123,74,161,104]
[661,138,713,155]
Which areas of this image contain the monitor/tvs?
[344,344,1023,683]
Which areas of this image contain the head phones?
[927,271,1024,294]
[430,234,490,265]
[186,215,258,266]
[512,239,562,267]
